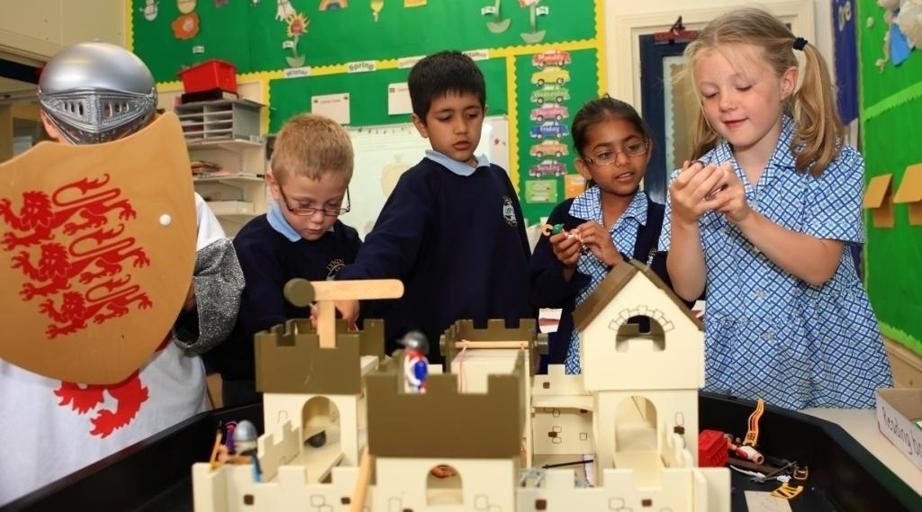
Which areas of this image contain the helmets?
[37,38,159,147]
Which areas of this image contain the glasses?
[278,182,351,217]
[579,140,647,166]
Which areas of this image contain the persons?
[308,50,530,365]
[1,40,247,507]
[532,96,666,372]
[658,9,893,409]
[219,116,365,430]
[211,419,265,484]
[400,326,430,394]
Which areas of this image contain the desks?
[798,407,922,512]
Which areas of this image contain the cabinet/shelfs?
[172,95,268,237]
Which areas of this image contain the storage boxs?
[177,60,237,93]
